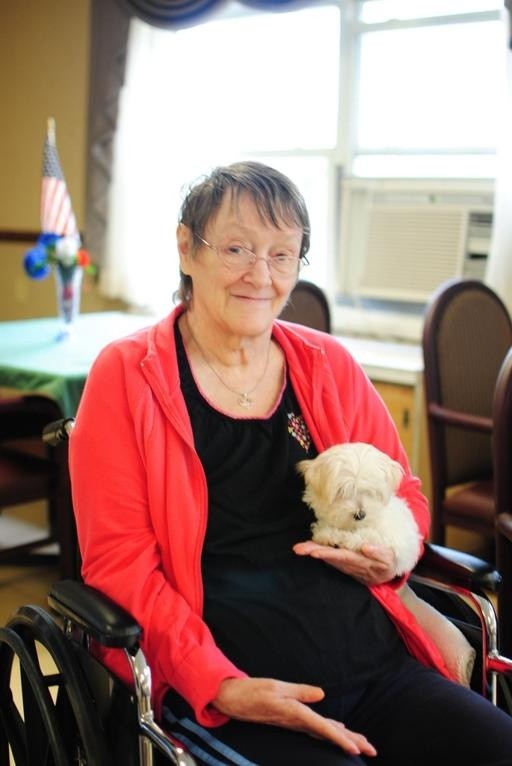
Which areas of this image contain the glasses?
[195,232,310,274]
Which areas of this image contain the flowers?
[23,233,99,323]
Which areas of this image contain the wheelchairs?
[0,418,512,766]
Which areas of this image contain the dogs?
[296,439,425,579]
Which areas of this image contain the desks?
[0,310,159,581]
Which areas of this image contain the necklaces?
[184,309,272,411]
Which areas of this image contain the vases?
[53,266,86,344]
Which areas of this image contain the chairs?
[276,280,330,334]
[421,278,512,660]
[0,394,63,507]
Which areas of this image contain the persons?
[68,161,512,766]
